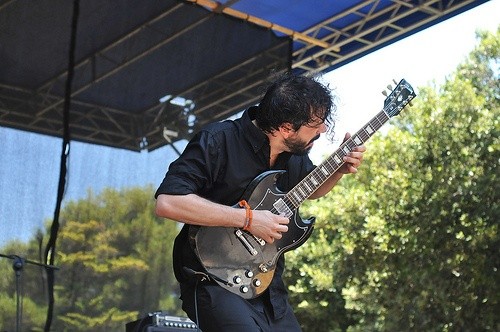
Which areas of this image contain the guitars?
[188,78,416,299]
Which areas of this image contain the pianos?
[126,311,198,332]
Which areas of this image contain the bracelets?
[238,199,253,230]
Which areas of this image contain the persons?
[156,73,365,331]
